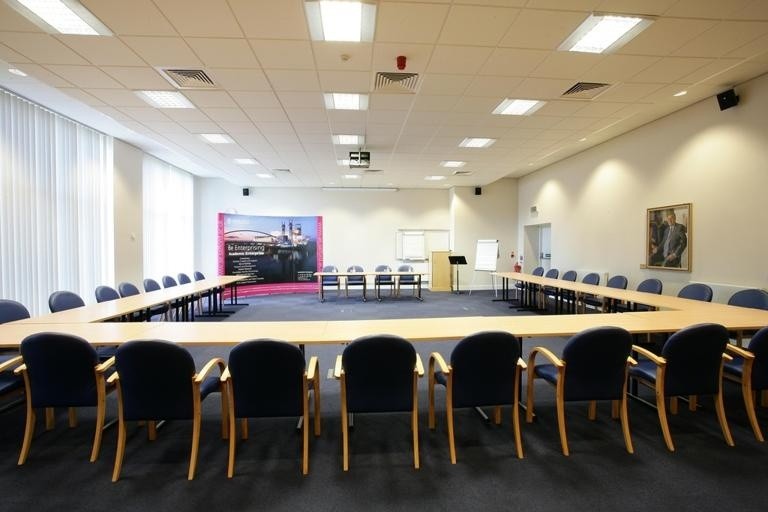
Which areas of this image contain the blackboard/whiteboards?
[396,228,451,262]
[474,239,499,272]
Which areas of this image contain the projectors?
[349,159,369,168]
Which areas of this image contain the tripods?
[450,263,465,295]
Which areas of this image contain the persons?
[652,209,687,246]
[648,211,687,268]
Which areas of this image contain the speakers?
[717,89,738,111]
[243,189,249,195]
[476,188,481,195]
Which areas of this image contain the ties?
[663,226,674,257]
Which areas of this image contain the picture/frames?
[646,201,693,273]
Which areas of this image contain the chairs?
[0,272,251,324]
[0,332,427,482]
[516,267,768,348]
[308,262,431,303]
[427,323,767,465]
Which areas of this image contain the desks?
[0,311,768,366]
[491,271,768,311]
